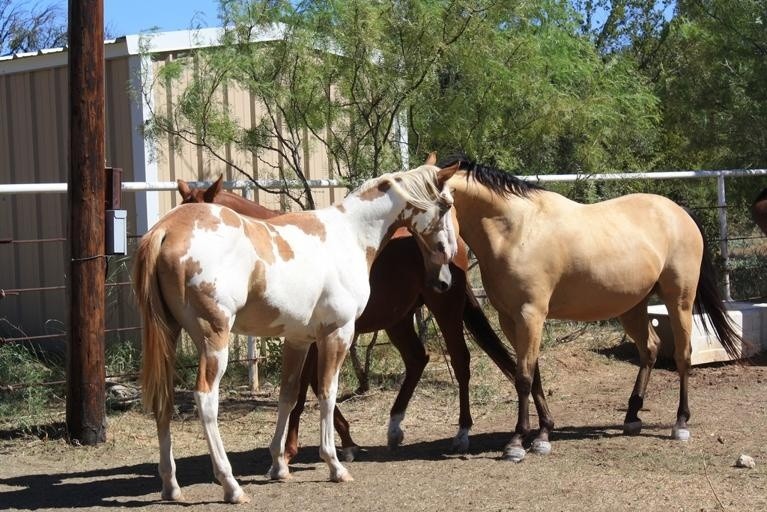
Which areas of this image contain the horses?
[175,174,517,464]
[440,152,751,462]
[129,149,461,505]
